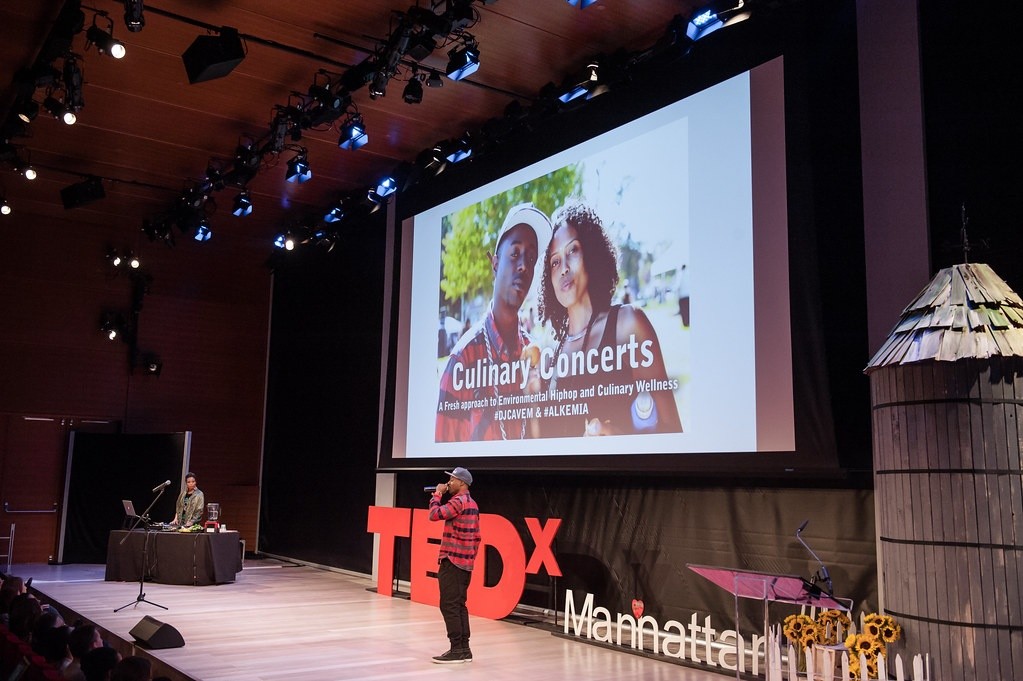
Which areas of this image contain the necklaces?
[563,317,597,341]
[482,314,526,440]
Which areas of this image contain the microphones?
[797,519,833,597]
[153,480,171,492]
[424,485,449,493]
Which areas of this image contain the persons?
[169,473,204,527]
[0,572,172,681]
[429,467,481,664]
[517,203,683,438]
[435,205,553,443]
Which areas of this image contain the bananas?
[178,529,191,532]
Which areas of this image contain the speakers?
[128,615,185,649]
[182,34,245,85]
[60,178,106,209]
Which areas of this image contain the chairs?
[0,621,67,681]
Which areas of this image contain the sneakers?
[431,649,465,664]
[462,651,473,661]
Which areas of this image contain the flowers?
[844,613,902,681]
[784,607,851,672]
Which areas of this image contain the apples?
[525,341,541,366]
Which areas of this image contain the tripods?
[113,488,168,613]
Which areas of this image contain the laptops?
[122,499,151,520]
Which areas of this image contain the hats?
[445,466,473,487]
[494,202,553,257]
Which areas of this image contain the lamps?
[98,304,164,376]
[1,2,751,275]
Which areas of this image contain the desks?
[105,529,242,587]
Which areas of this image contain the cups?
[221,524,226,531]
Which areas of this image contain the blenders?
[204,503,219,533]
